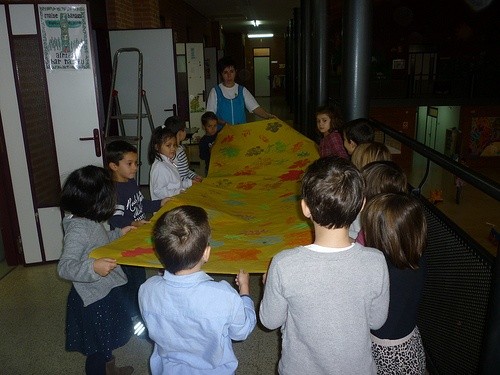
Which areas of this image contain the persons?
[138,204,257,375]
[206,57,278,131]
[164,115,204,192]
[360,194,427,375]
[259,157,389,375]
[56,165,138,375]
[316,105,351,161]
[148,126,202,275]
[344,118,409,246]
[103,141,179,339]
[198,112,223,176]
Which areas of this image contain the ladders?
[103,48,158,186]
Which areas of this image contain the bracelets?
[223,121,227,126]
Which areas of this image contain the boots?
[105,355,135,375]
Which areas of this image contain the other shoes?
[131,315,149,340]
